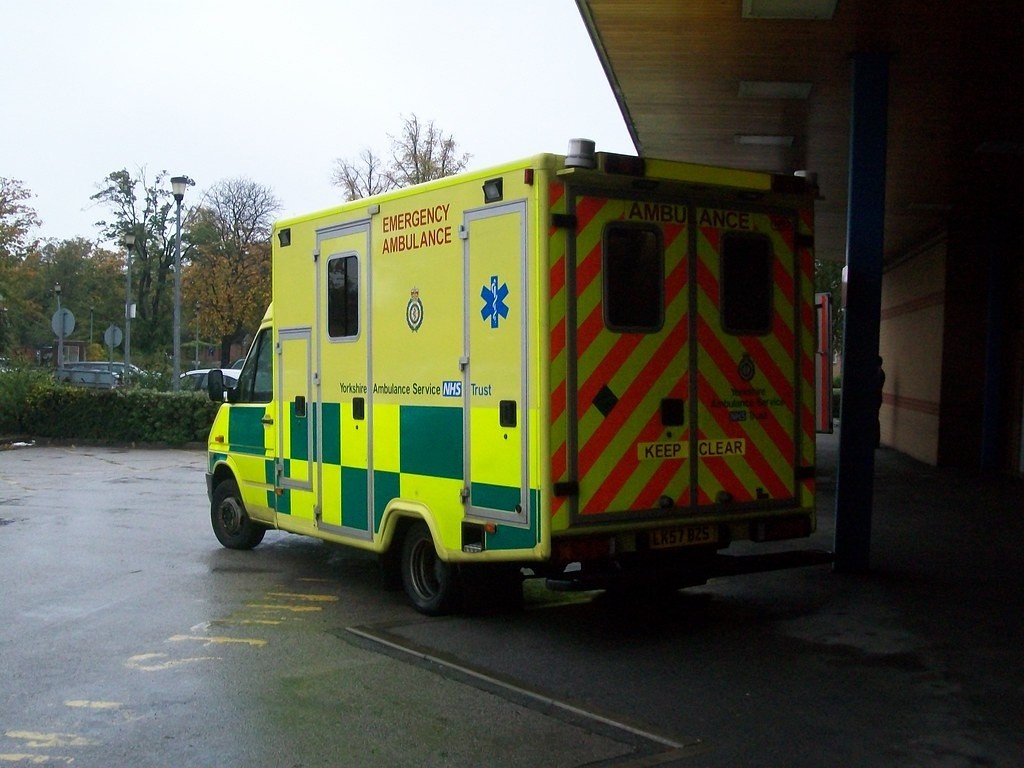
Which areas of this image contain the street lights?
[89,302,95,347]
[123,229,136,380]
[54,281,61,310]
[170,176,187,392]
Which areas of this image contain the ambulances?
[203,138,819,619]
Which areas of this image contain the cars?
[179,358,246,390]
[59,359,148,390]
